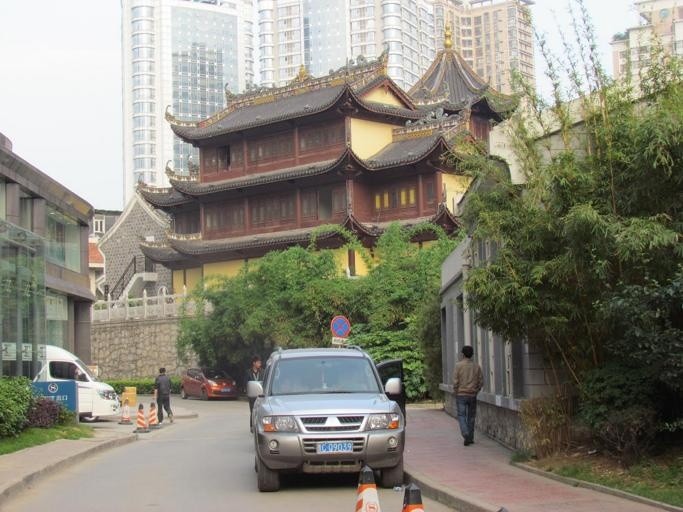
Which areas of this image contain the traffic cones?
[354,466,425,512]
[118,399,161,433]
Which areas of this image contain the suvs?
[181,367,239,400]
[248,345,408,490]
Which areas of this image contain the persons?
[240,353,266,435]
[451,344,484,446]
[150,367,174,424]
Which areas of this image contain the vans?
[1,342,120,422]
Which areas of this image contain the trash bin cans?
[376,358,406,427]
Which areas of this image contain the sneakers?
[463,434,475,446]
